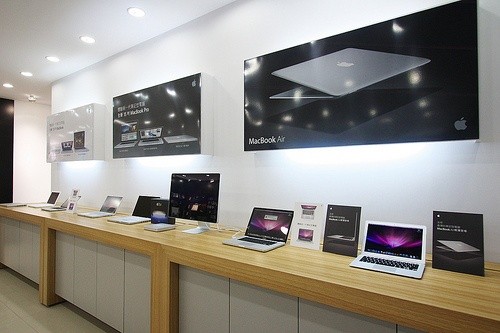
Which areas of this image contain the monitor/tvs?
[167,173,220,235]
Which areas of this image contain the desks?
[0,203,500,333]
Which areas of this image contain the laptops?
[78,195,124,219]
[349,220,427,279]
[114,122,198,149]
[27,191,81,212]
[269,48,431,99]
[50,131,88,154]
[222,208,294,253]
[436,240,480,253]
[108,196,160,225]
[296,228,314,244]
[326,212,357,241]
